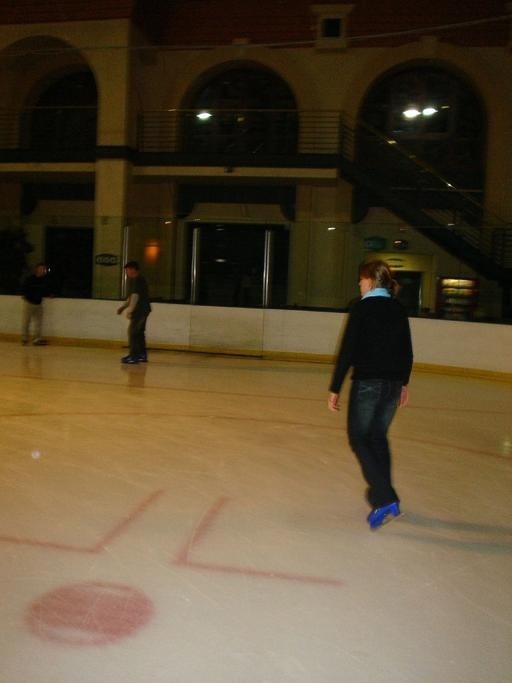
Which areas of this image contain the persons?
[117,261,152,363]
[329,259,412,527]
[21,263,50,345]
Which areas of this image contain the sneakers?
[122,352,146,363]
[368,500,399,526]
[33,339,47,344]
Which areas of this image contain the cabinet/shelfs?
[437,276,478,321]
